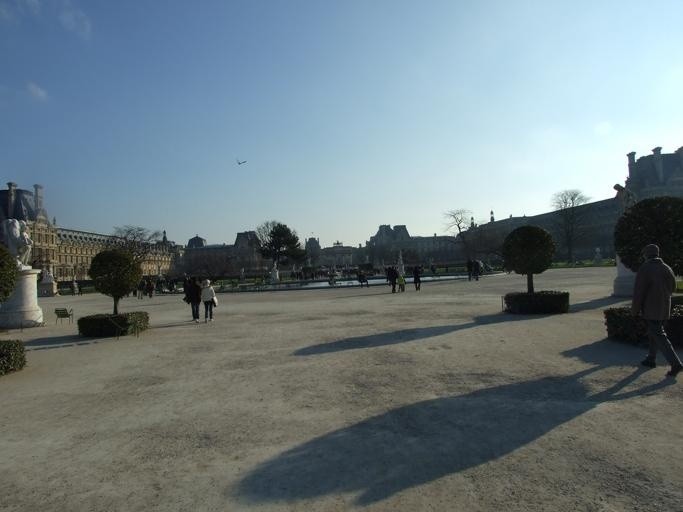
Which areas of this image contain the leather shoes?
[667,362,682,376]
[640,358,656,368]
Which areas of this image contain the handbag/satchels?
[212,296,217,307]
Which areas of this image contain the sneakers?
[191,318,214,324]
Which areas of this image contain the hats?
[641,244,659,256]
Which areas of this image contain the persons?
[136,271,216,324]
[630,243,682,377]
[289,258,483,294]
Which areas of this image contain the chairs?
[54,307,73,326]
